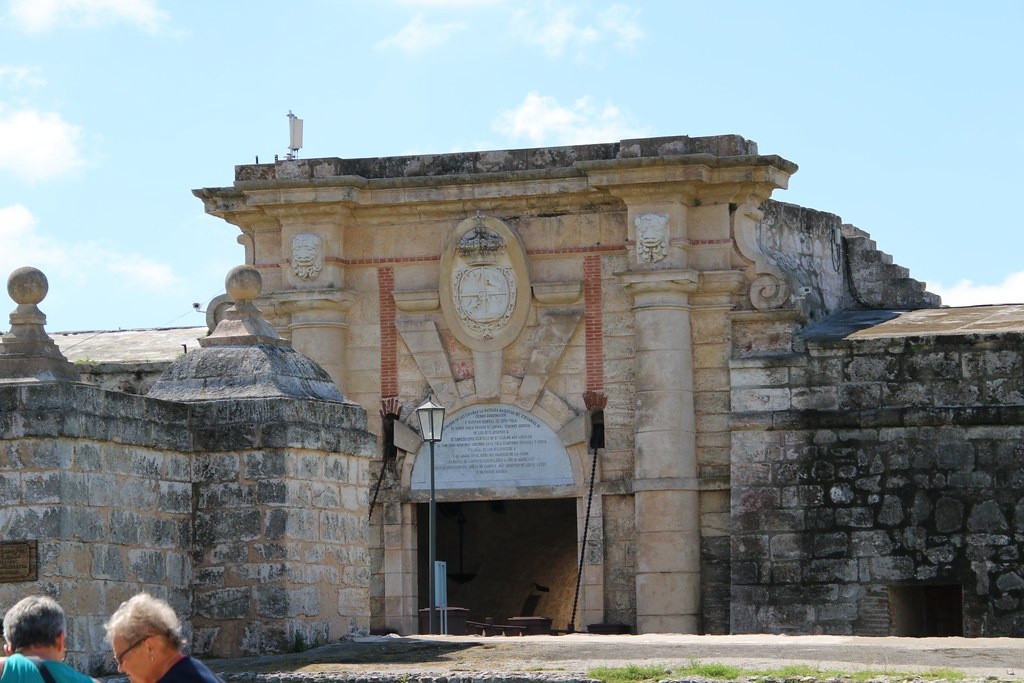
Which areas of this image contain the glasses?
[113,635,151,664]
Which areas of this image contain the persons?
[0,596,102,683]
[103,592,222,683]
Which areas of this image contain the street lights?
[415,395,447,635]
